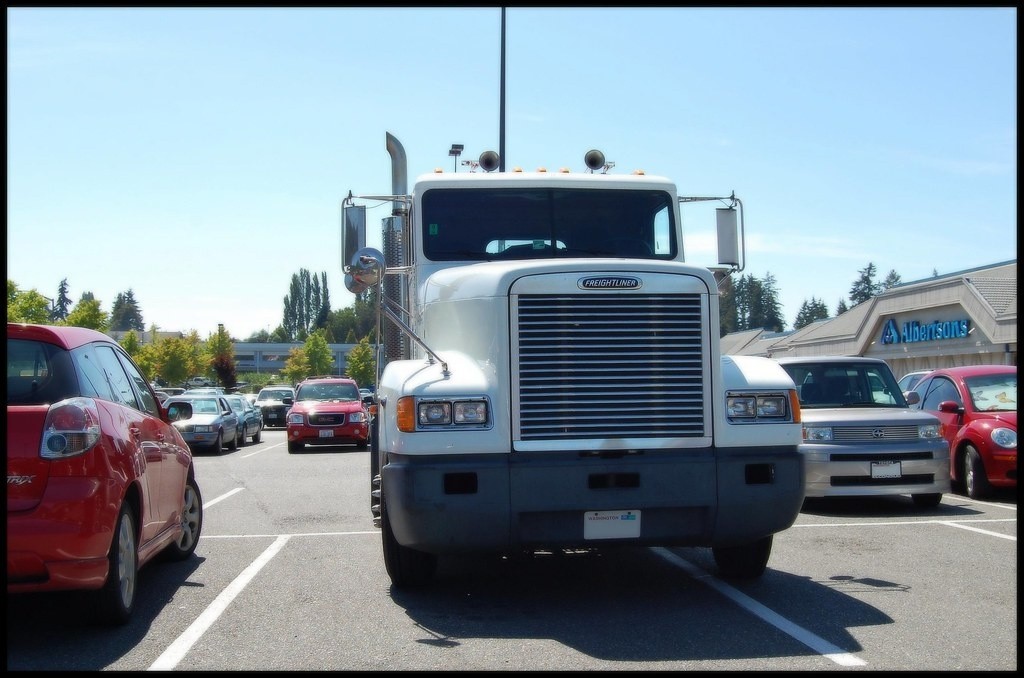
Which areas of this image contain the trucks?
[338,128,809,589]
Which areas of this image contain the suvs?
[776,355,952,513]
[282,374,374,455]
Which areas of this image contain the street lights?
[449,143,464,174]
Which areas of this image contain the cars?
[151,376,297,457]
[883,369,934,401]
[5,321,203,624]
[797,371,896,405]
[359,384,375,410]
[909,364,1017,502]
